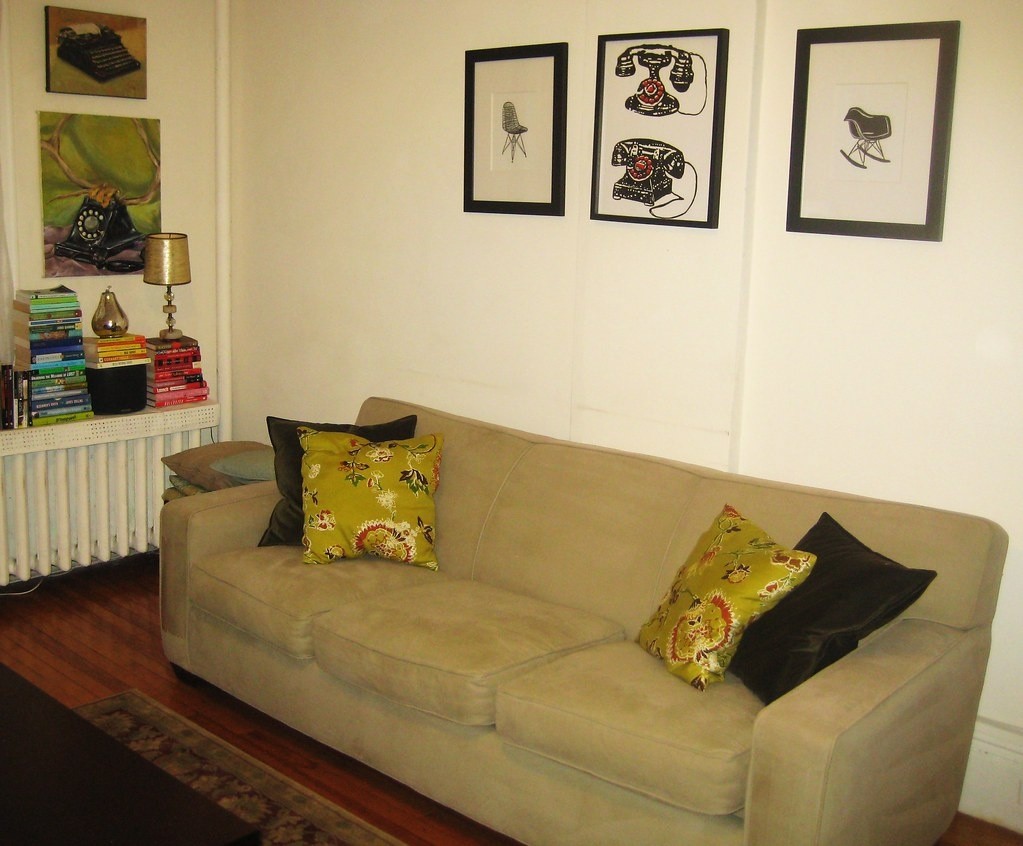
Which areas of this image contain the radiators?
[0,400,220,587]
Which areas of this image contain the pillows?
[727,511,937,707]
[636,504,816,694]
[257,415,418,546]
[297,426,445,572]
[161,439,273,502]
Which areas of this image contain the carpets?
[72,689,408,846]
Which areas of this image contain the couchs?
[158,396,1009,846]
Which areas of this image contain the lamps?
[143,232,191,339]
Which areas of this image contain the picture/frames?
[463,42,568,217]
[590,28,730,228]
[786,20,960,242]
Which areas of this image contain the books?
[145,335,211,408]
[12,285,94,427]
[83,333,152,370]
[0,361,29,431]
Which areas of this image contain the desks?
[0,661,261,846]
